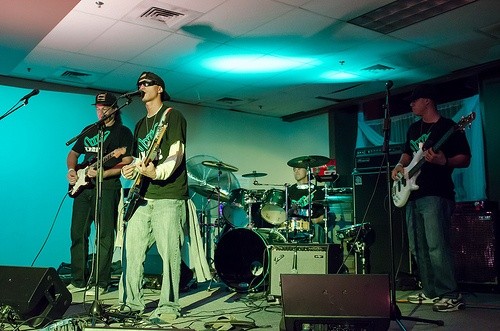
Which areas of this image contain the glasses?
[138,82,158,87]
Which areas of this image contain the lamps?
[185,154,232,291]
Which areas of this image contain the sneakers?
[407,292,441,304]
[433,294,464,311]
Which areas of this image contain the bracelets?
[69,168,74,172]
[397,163,403,167]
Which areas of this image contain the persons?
[391,85,472,311]
[288,167,330,225]
[105,71,191,318]
[66,91,133,295]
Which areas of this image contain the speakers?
[0,266,72,328]
[448,212,500,288]
[268,242,392,331]
[352,171,413,275]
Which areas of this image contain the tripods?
[383,88,444,331]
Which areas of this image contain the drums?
[220,188,262,228]
[336,222,372,239]
[213,227,289,293]
[260,189,291,226]
[280,216,313,238]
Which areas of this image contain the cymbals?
[314,200,328,205]
[189,183,229,202]
[287,154,331,169]
[202,160,238,172]
[242,173,267,178]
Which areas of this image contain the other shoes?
[67,283,88,293]
[150,309,178,323]
[85,285,106,296]
[107,303,145,315]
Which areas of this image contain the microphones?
[121,89,144,98]
[20,89,40,101]
[291,248,298,274]
[384,80,393,89]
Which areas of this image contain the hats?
[407,87,435,102]
[92,93,117,108]
[137,71,170,101]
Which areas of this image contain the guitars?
[121,122,168,223]
[391,111,476,208]
[68,144,128,198]
